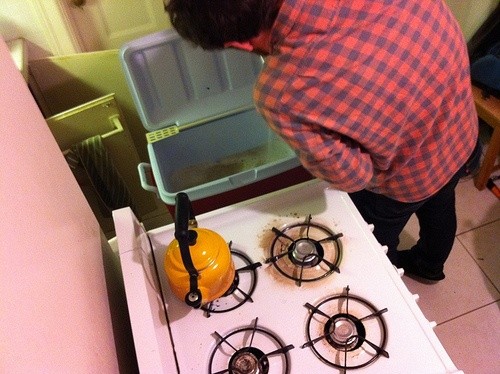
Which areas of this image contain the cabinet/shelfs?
[21,38,158,235]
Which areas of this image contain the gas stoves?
[111,177,464,374]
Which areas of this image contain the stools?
[468,79,500,191]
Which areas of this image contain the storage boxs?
[118,25,317,224]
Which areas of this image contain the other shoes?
[388,249,441,285]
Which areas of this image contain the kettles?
[164,193,235,311]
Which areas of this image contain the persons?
[163,1,480,281]
